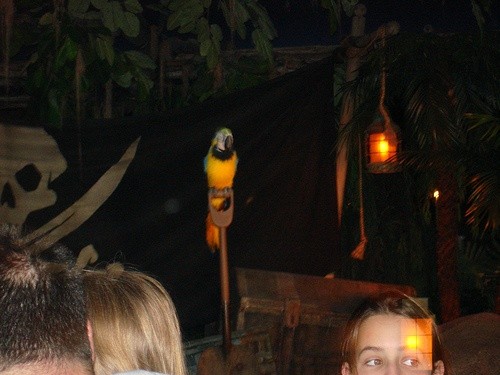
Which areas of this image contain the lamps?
[362,106,407,175]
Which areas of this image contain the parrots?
[203,127,238,252]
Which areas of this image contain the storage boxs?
[227,268,419,375]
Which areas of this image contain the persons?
[81,268,188,375]
[336,294,447,375]
[0,223,99,375]
